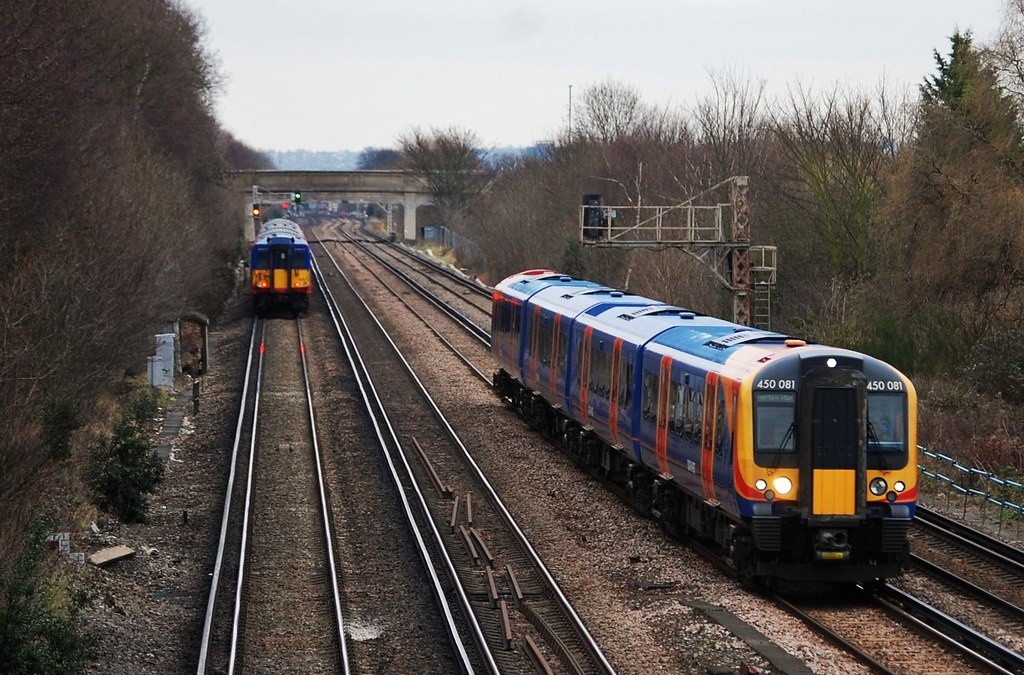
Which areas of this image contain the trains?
[491,268,916,604]
[250,218,312,322]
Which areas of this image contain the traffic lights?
[281,201,288,209]
[254,204,261,219]
[295,191,301,204]
